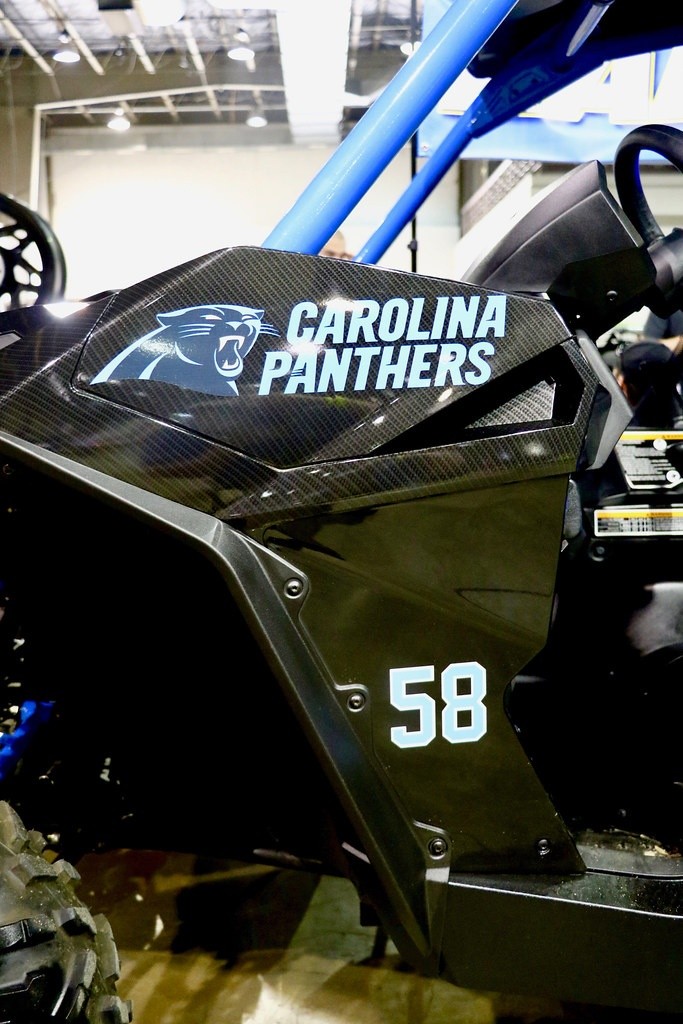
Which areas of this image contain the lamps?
[247,101,268,127]
[229,35,255,59]
[54,31,80,63]
